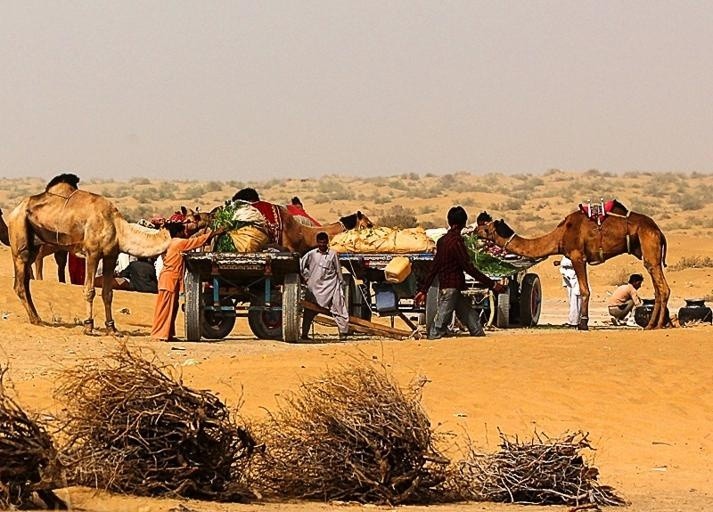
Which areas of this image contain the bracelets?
[492,280,497,289]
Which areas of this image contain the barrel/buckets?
[383,255,413,283]
[374,285,397,312]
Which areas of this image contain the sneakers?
[611,317,626,326]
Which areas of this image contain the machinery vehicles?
[335,251,541,328]
[184,252,299,342]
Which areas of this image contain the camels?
[472,204,673,331]
[5,180,216,339]
[234,198,376,340]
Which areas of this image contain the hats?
[169,223,186,230]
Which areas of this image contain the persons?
[551,250,590,328]
[115,255,157,293]
[476,212,492,227]
[410,205,506,340]
[604,272,644,328]
[148,222,226,342]
[298,230,353,344]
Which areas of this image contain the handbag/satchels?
[230,225,268,252]
